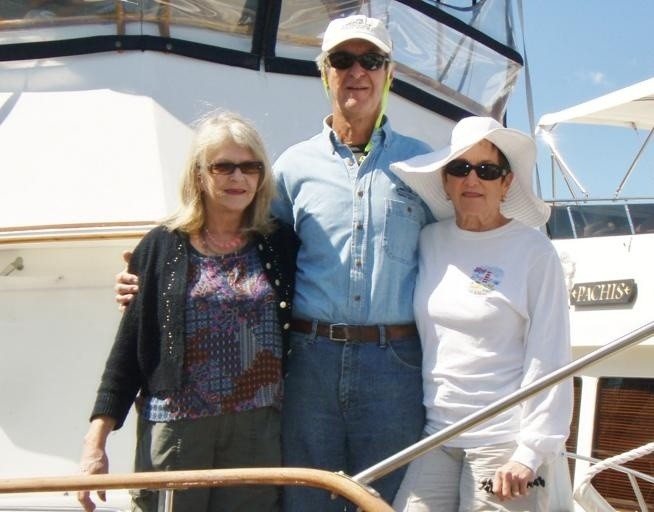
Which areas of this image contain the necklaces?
[199,222,246,250]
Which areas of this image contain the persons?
[387,115,575,512]
[76,106,298,512]
[114,15,432,512]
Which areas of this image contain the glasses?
[445,159,507,181]
[198,161,264,175]
[325,51,391,71]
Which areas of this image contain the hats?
[388,116,551,227]
[321,15,393,60]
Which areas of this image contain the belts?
[285,315,416,344]
